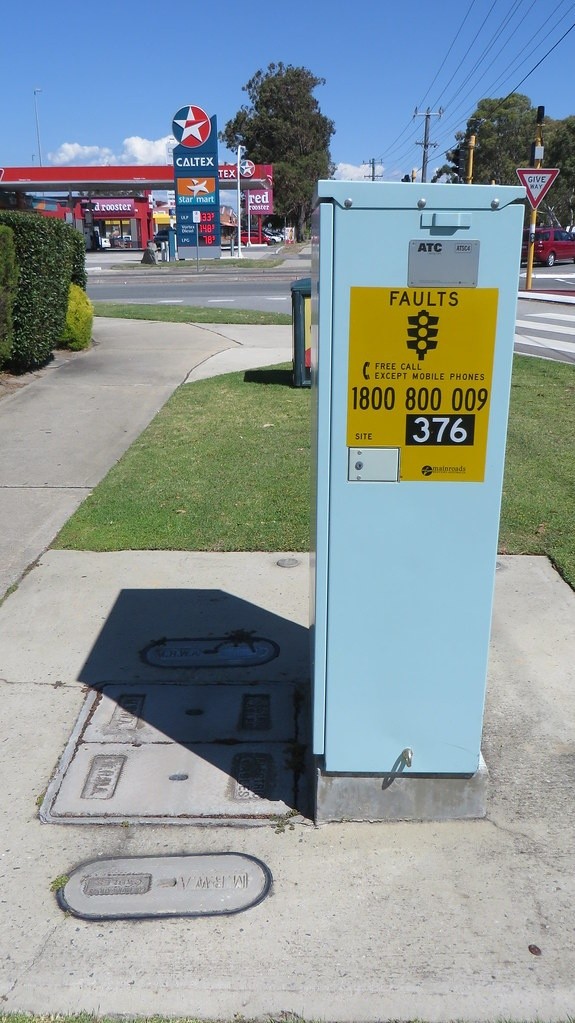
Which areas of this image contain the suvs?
[521,227,575,267]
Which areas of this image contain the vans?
[118,234,131,241]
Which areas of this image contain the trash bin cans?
[290,277,311,388]
[168,229,178,262]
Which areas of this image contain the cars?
[153,230,169,248]
[234,225,285,246]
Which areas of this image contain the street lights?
[34,89,43,167]
[237,145,246,258]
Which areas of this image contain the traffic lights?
[451,148,466,177]
